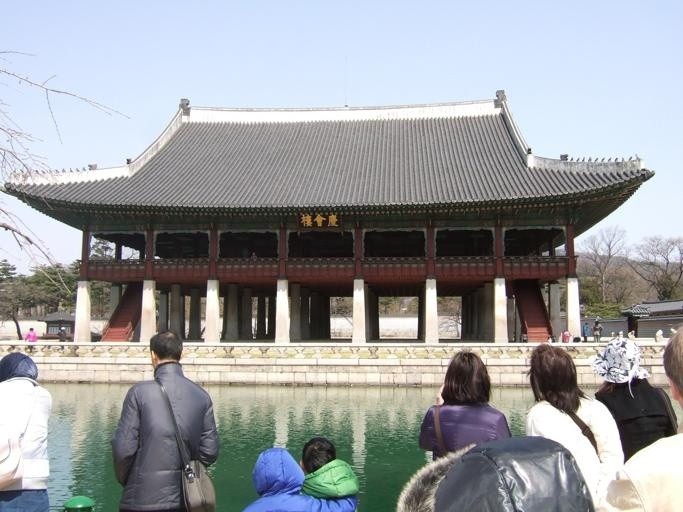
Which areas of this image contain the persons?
[0,352,52,512]
[417,350,510,461]
[606,326,683,512]
[545,329,664,343]
[241,447,356,512]
[298,438,336,475]
[525,343,625,512]
[57,327,66,349]
[591,336,677,465]
[394,436,595,512]
[23,327,36,353]
[112,330,218,512]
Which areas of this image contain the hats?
[592,336,652,385]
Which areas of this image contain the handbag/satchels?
[0,438,24,490]
[180,460,216,512]
